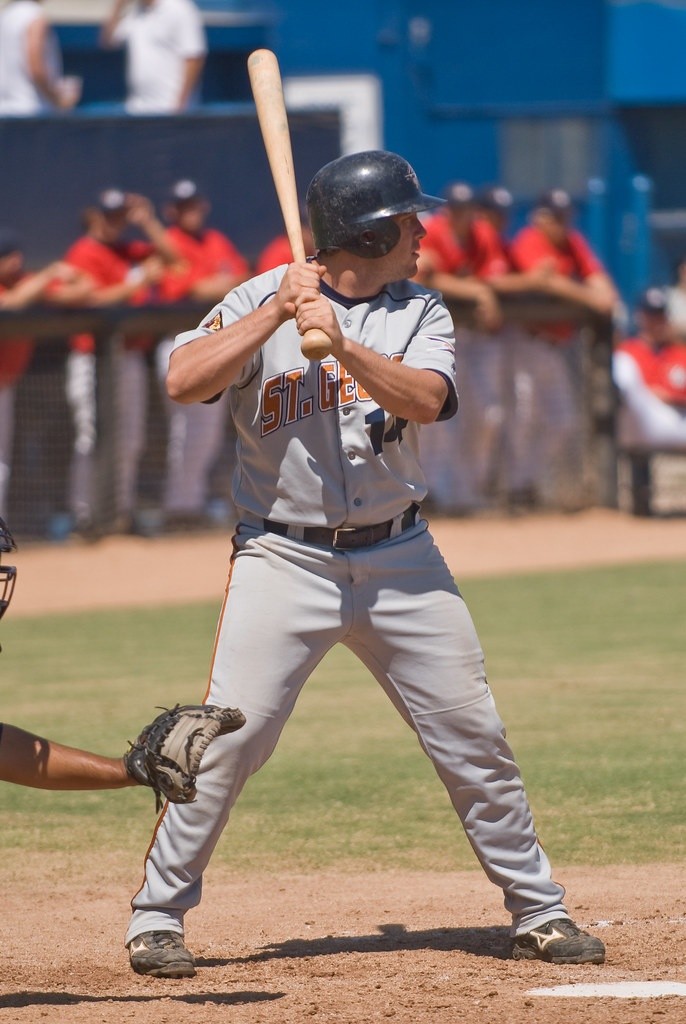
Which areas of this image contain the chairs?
[615,406,686,518]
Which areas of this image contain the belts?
[262,503,417,551]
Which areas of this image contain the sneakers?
[512,918,605,965]
[128,930,194,976]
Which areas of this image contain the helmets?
[305,150,447,259]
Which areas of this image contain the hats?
[448,184,476,211]
[484,185,513,209]
[174,181,202,202]
[642,287,668,315]
[99,189,127,213]
[541,184,570,210]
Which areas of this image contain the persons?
[0,230,101,529]
[124,154,606,978]
[407,185,516,518]
[258,196,318,274]
[0,705,246,803]
[613,286,686,454]
[2,0,207,114]
[506,187,619,513]
[149,180,252,532]
[62,189,190,551]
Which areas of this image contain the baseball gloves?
[123,704,246,804]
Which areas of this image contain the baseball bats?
[246,48,333,360]
